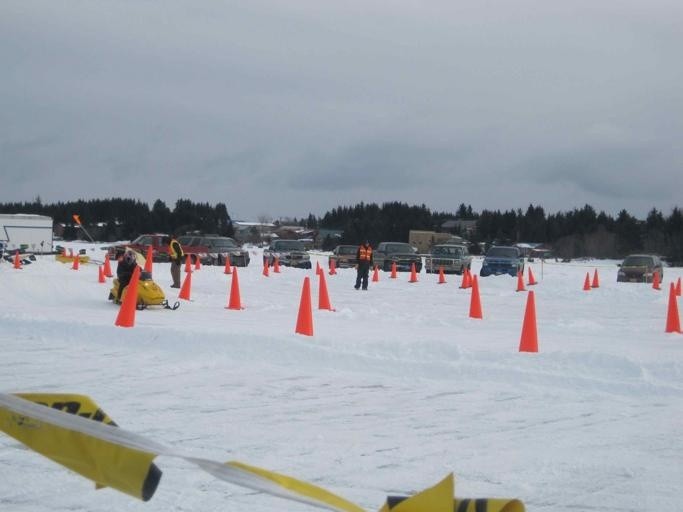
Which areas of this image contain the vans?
[615,255,666,284]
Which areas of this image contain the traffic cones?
[11,251,22,271]
[59,248,82,271]
[674,277,683,295]
[665,281,682,334]
[647,271,660,291]
[590,267,602,288]
[582,269,593,292]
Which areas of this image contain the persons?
[164,237,185,288]
[354,240,375,290]
[113,252,143,305]
